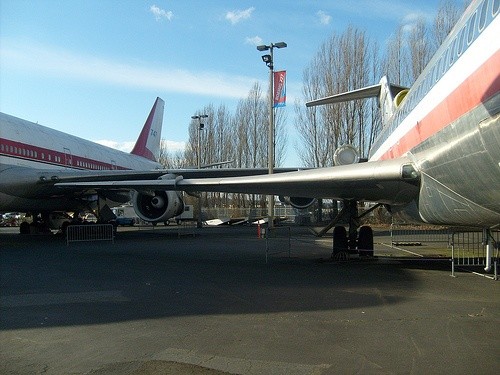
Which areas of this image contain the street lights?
[191,114,209,226]
[257,42,288,238]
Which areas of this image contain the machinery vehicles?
[110,201,194,227]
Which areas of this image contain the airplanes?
[47,0,500,265]
[0,97,186,247]
